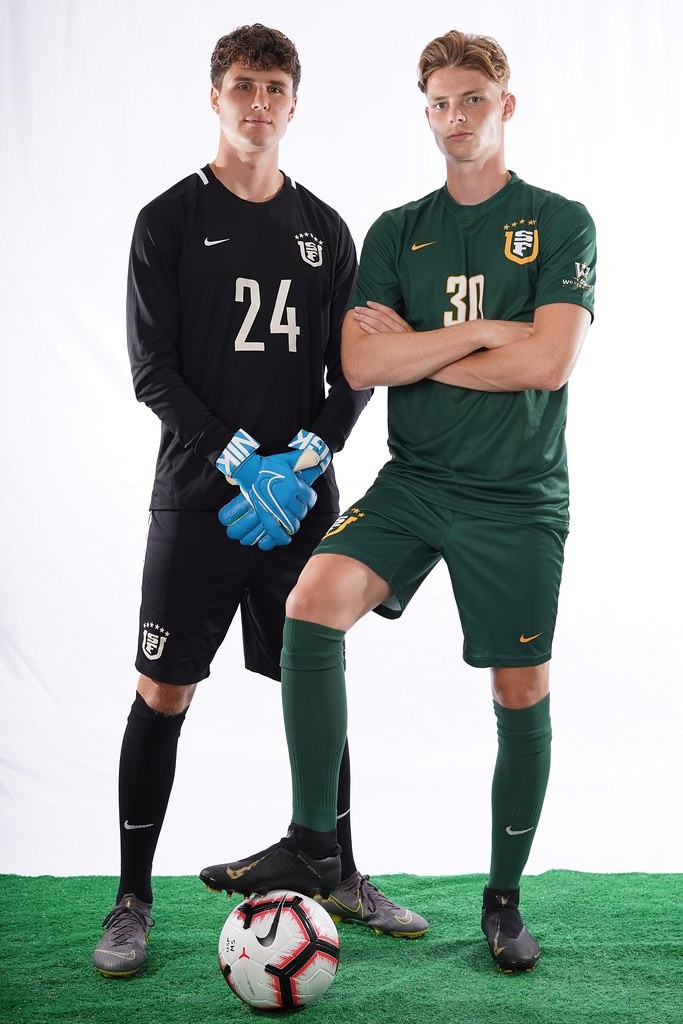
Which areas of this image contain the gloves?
[216,428,332,550]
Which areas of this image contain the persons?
[194,32,602,978]
[89,23,434,978]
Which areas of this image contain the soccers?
[216,888,339,1013]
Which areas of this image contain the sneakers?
[312,871,429,939]
[200,837,342,903]
[482,884,541,973]
[94,893,153,979]
